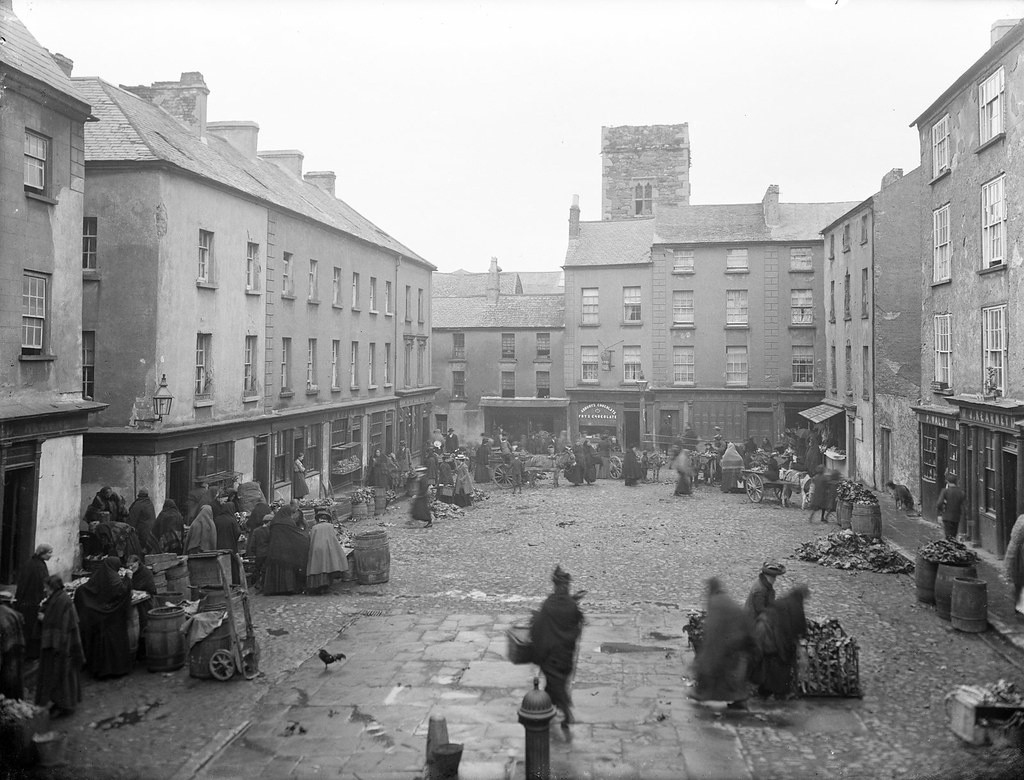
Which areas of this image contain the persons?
[936,473,965,539]
[0,476,349,720]
[369,426,473,528]
[669,423,840,522]
[474,424,662,494]
[294,453,308,498]
[690,562,810,709]
[888,482,914,509]
[528,567,584,726]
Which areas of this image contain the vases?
[365,500,376,517]
[272,506,281,515]
[301,508,315,530]
[351,503,367,520]
[317,506,335,519]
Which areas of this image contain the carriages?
[487,445,578,490]
[607,446,670,484]
[740,461,815,511]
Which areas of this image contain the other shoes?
[47,702,72,719]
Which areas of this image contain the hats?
[760,559,787,576]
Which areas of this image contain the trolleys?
[212,554,262,682]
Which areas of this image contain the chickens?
[318,647,346,669]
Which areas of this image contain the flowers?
[348,486,376,503]
[233,510,249,527]
[269,495,335,511]
[384,488,396,501]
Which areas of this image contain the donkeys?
[646,451,667,484]
[781,468,814,510]
[688,451,703,490]
[528,451,577,487]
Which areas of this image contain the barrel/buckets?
[353,529,390,585]
[934,563,978,620]
[124,552,236,681]
[835,495,882,539]
[351,485,388,520]
[722,459,747,493]
[914,546,951,602]
[949,578,988,634]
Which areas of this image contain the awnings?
[798,404,844,424]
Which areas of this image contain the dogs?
[885,481,914,512]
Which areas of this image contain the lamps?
[134,374,175,426]
